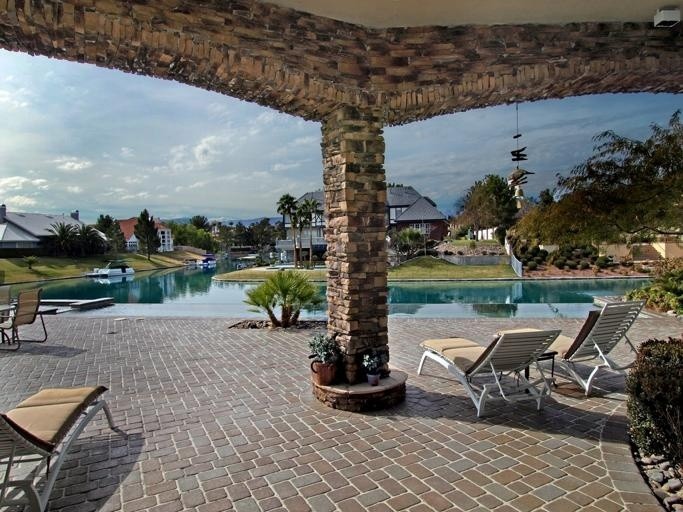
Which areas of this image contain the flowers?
[362,350,387,374]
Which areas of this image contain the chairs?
[0,288,47,352]
[0,386,115,512]
[0,286,16,343]
[491,298,649,396]
[417,328,562,417]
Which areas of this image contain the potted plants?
[306,332,339,385]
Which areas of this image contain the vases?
[367,374,380,387]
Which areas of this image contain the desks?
[0,304,17,343]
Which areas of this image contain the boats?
[235,263,247,269]
[187,264,217,275]
[90,275,134,285]
[86,260,135,277]
[182,252,218,265]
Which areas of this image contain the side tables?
[522,347,559,394]
